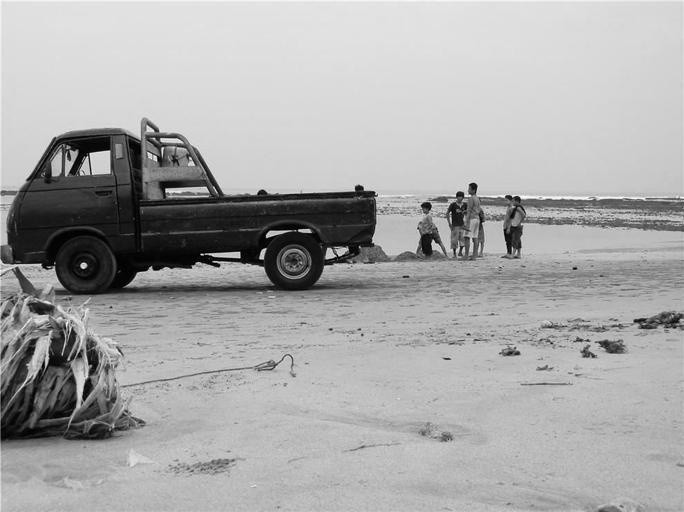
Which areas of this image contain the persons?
[458,183,480,260]
[415,191,525,261]
[354,184,374,248]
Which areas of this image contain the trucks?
[0,118,376,294]
